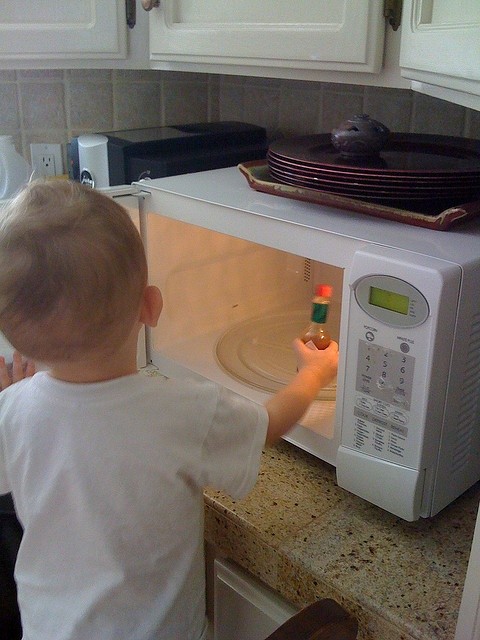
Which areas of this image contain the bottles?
[300,285,333,383]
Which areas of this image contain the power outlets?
[28,140,63,179]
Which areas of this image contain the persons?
[0,177,339,640]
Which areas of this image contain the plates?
[264,134,475,212]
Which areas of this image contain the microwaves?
[0,150,474,519]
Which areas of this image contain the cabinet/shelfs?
[0,0,409,91]
[398,0,480,112]
